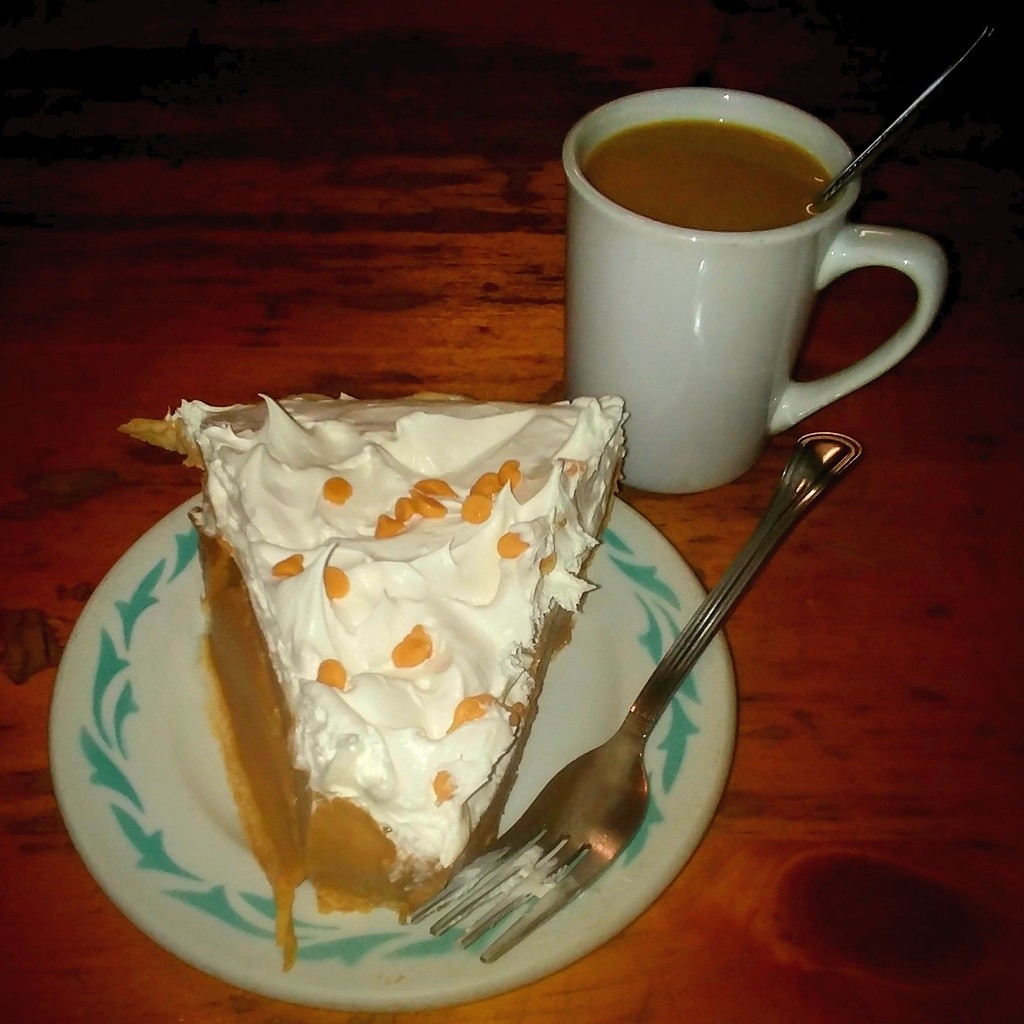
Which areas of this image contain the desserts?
[118,389,631,968]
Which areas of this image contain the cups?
[562,87,945,494]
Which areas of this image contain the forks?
[407,431,862,962]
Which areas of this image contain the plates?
[51,487,737,1011]
